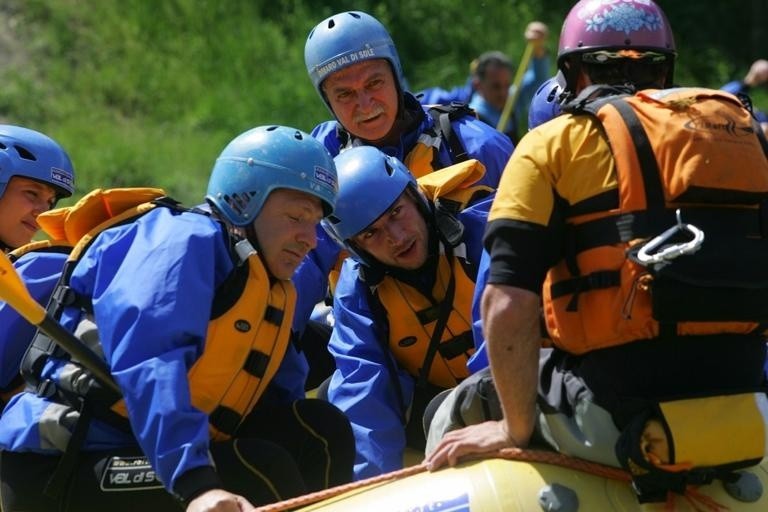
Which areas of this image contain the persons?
[523,73,569,131]
[720,60,768,143]
[423,0,768,482]
[306,10,514,275]
[325,146,502,480]
[0,124,74,392]
[0,123,339,512]
[463,22,549,148]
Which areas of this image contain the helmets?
[321,145,430,268]
[0,125,74,209]
[304,11,404,130]
[205,124,338,227]
[527,0,678,131]
